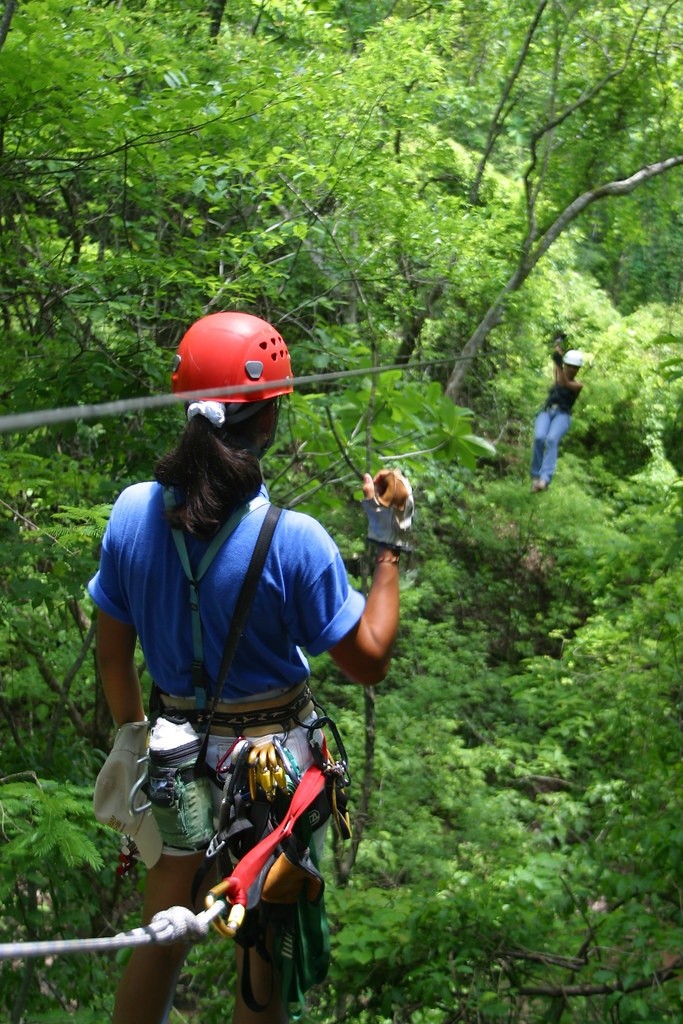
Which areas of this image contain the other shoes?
[530,482,538,493]
[533,482,545,490]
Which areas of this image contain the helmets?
[171,313,292,403]
[563,351,581,367]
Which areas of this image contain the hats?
[92,721,163,869]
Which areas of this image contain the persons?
[529,344,584,494]
[88,313,415,1023]
